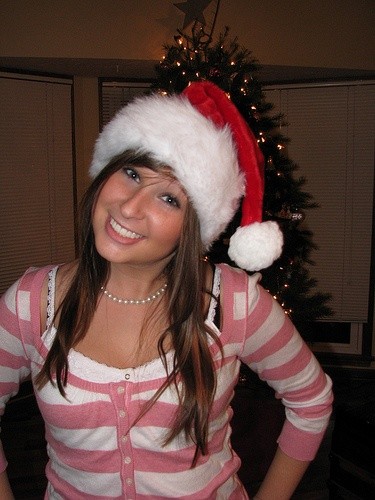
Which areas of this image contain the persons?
[0,80,334,499]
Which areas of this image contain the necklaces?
[99,279,168,305]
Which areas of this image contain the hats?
[90,81,284,271]
